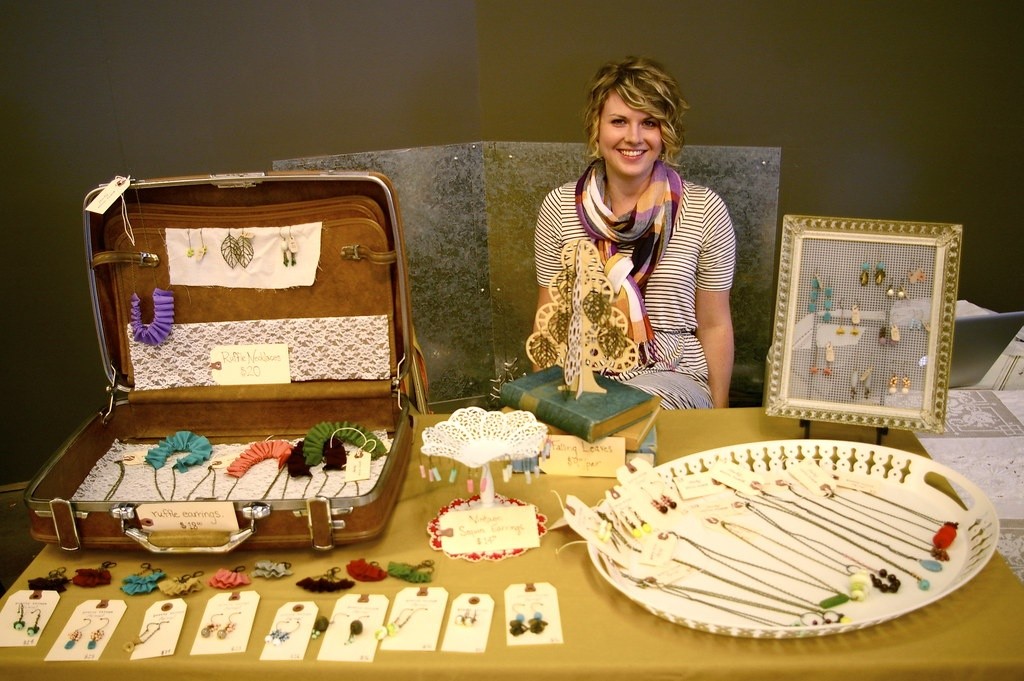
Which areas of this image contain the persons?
[498,51,736,437]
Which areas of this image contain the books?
[500,365,663,478]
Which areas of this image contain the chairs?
[273,142,501,411]
[488,139,784,408]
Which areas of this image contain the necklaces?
[116,178,175,345]
[104,419,386,507]
[615,475,961,630]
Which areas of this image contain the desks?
[0,392,1024,681]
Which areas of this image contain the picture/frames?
[766,214,963,433]
